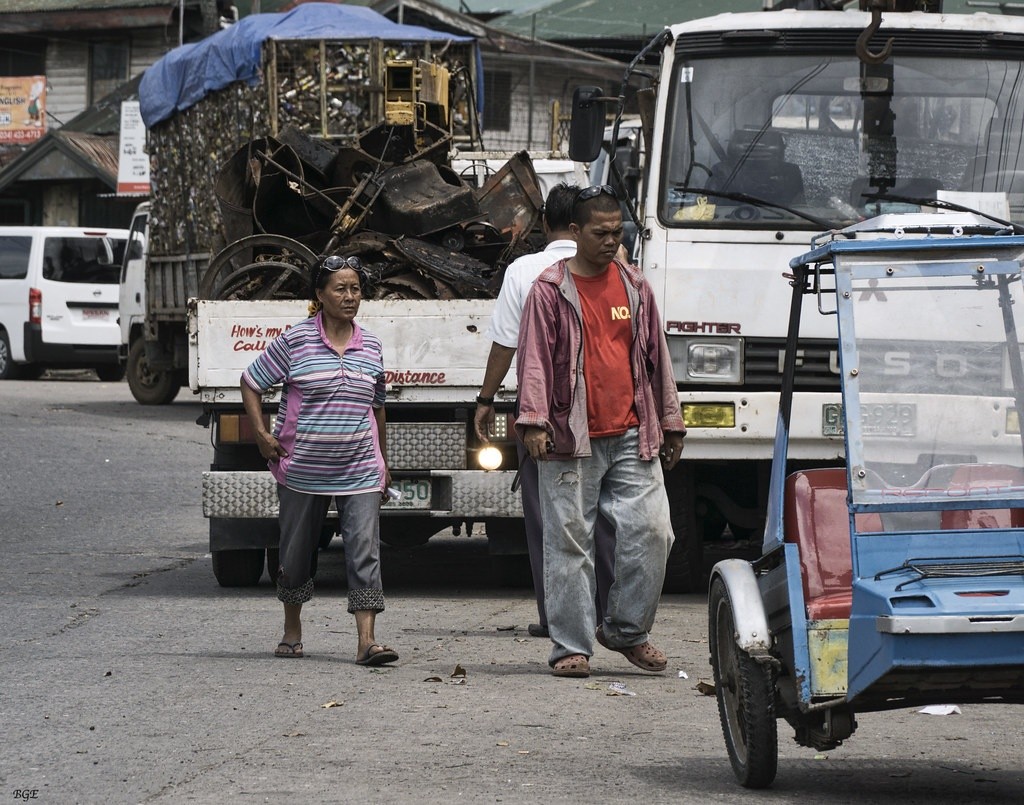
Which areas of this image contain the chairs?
[959,117,1024,193]
[53,245,85,280]
[701,128,809,207]
[942,465,1024,530]
[787,468,885,617]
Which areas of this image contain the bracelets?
[476,396,494,406]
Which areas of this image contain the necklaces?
[325,324,353,354]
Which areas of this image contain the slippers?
[355,643,398,665]
[274,643,304,658]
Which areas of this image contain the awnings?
[485,0,764,39]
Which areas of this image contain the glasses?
[538,207,548,218]
[313,255,364,285]
[575,184,618,207]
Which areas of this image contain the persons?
[474,185,687,678]
[239,257,400,665]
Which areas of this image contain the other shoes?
[528,623,550,637]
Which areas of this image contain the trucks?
[96,37,474,418]
[186,159,592,587]
[567,0,1024,594]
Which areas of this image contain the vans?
[1,224,145,383]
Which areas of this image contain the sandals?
[596,624,667,671]
[552,653,591,677]
